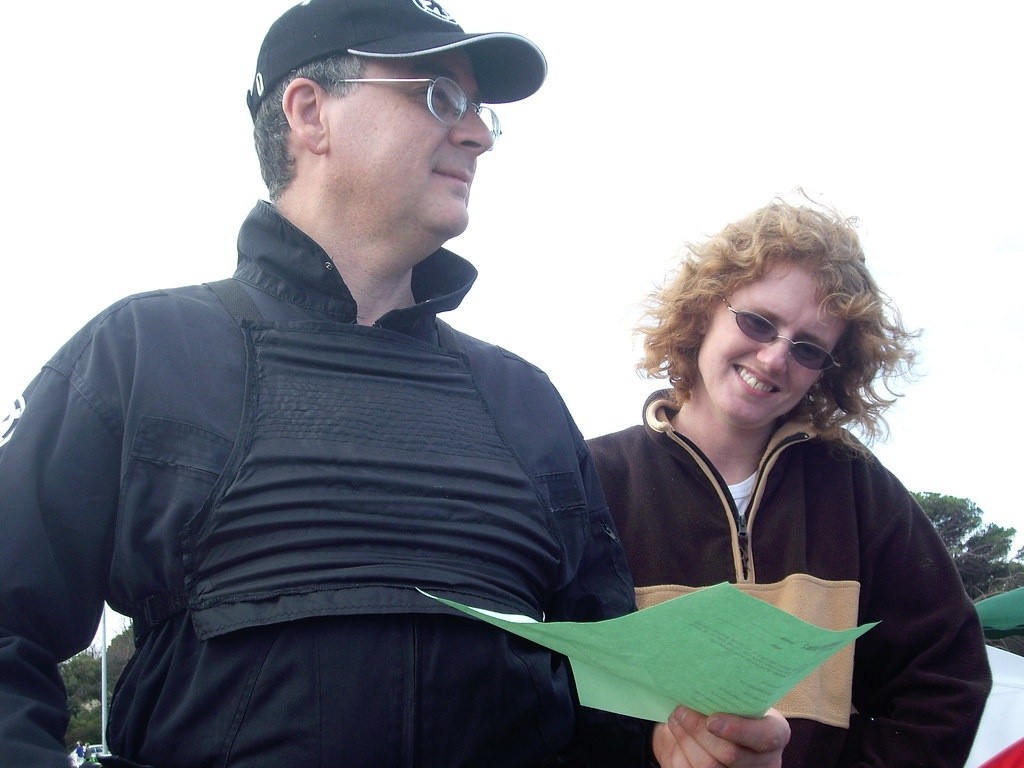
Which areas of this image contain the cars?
[68,744,113,768]
[965,645,1024,768]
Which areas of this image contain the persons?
[581,188,991,768]
[83,743,91,762]
[0,0,791,768]
[74,741,83,767]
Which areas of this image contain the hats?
[247,0,548,124]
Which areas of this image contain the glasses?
[719,293,842,372]
[336,77,503,150]
